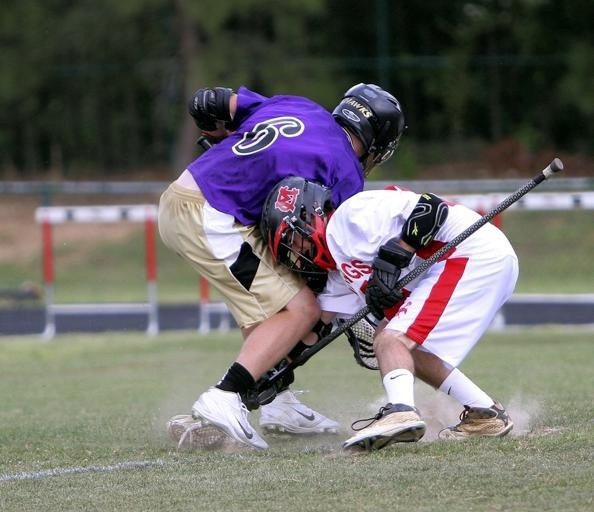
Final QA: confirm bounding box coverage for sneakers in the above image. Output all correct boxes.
[435,401,513,443]
[191,386,270,451]
[340,404,426,452]
[259,388,342,436]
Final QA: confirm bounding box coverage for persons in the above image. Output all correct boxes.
[259,177,519,452]
[157,83,404,452]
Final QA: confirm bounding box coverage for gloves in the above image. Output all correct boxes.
[364,238,412,321]
[240,358,294,410]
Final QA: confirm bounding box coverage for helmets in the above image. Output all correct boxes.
[260,176,334,285]
[332,82,407,176]
